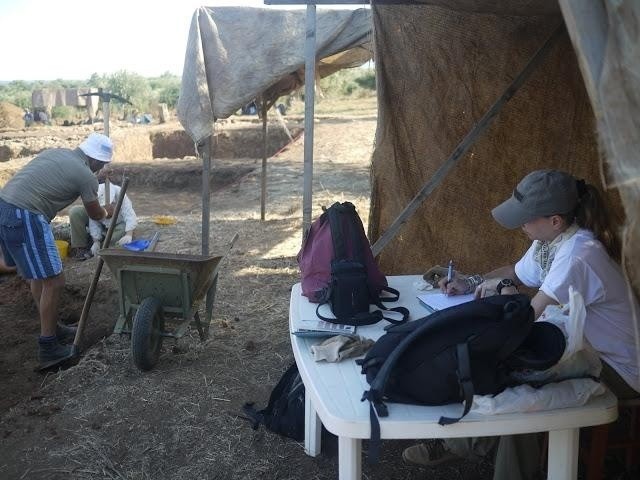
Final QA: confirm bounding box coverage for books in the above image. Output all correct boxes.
[417,292,475,314]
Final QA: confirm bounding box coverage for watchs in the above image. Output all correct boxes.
[496,278,518,295]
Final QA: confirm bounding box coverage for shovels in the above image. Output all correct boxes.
[34,178,129,373]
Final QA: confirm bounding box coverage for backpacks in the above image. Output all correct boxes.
[251,361,305,440]
[297,201,409,326]
[356,294,566,426]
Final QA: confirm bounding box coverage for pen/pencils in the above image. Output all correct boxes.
[445,259,453,298]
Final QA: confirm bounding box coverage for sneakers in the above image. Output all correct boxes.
[402,440,456,467]
[37,325,78,366]
[73,248,88,261]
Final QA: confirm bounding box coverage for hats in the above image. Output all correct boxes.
[79,133,114,163]
[98,183,115,206]
[491,169,579,230]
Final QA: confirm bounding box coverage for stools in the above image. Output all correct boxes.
[536,395,640,479]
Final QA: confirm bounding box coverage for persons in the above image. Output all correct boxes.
[404,168,640,469]
[0,132,114,363]
[69,182,137,260]
[23,108,34,127]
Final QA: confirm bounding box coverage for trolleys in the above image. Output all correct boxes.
[97,229,242,373]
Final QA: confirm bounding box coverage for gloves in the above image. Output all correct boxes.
[117,231,133,245]
[90,241,101,256]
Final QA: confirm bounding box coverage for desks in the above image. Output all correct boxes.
[288,270,619,480]
